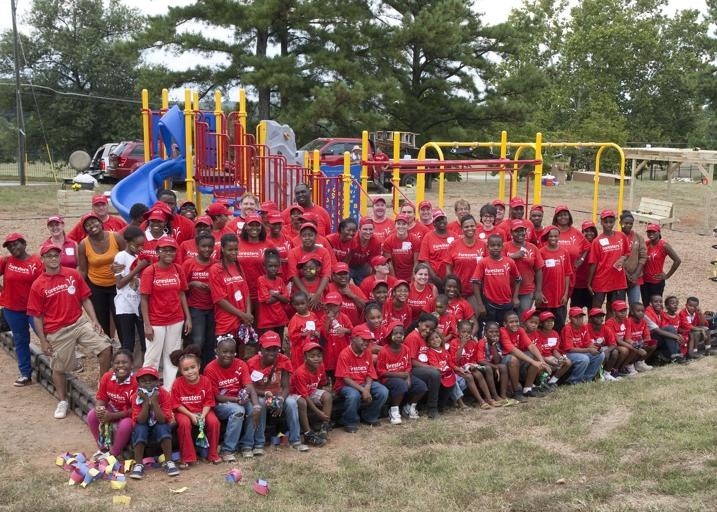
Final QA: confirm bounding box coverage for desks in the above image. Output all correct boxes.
[57,190,95,218]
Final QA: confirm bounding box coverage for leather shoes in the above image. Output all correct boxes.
[344,426,356,433]
[291,443,309,451]
[224,448,264,461]
[369,421,381,426]
[428,407,438,418]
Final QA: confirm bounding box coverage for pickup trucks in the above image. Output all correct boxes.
[300,137,440,192]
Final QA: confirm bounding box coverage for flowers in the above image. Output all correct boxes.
[72,183,81,188]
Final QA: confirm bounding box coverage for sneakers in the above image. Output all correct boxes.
[388,406,401,424]
[510,383,558,402]
[162,460,180,475]
[401,402,419,419]
[318,423,328,440]
[75,363,83,373]
[304,431,327,448]
[14,377,32,387]
[130,464,146,478]
[55,399,68,419]
[594,360,653,383]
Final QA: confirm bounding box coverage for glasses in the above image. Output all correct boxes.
[46,253,60,258]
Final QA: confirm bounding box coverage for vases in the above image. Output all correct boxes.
[73,187,80,191]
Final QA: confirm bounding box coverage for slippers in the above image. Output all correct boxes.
[461,398,519,412]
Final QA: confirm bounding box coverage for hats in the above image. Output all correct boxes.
[440,367,456,388]
[259,331,281,349]
[40,244,62,256]
[600,209,614,220]
[180,197,233,228]
[371,255,409,291]
[359,196,446,225]
[383,321,404,343]
[582,220,594,231]
[521,309,536,323]
[303,342,323,353]
[297,253,349,306]
[351,324,373,339]
[246,201,317,236]
[48,195,108,227]
[643,339,656,347]
[492,197,570,242]
[646,224,660,232]
[131,201,178,271]
[539,311,556,322]
[568,300,627,317]
[4,233,23,246]
[136,367,160,381]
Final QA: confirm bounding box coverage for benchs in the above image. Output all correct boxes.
[104,189,179,214]
[631,197,674,230]
[367,194,394,207]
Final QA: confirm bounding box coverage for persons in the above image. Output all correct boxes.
[350,145,362,165]
[171,144,179,158]
[372,148,389,194]
[1,184,711,479]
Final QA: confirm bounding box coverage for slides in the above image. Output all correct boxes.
[110,155,186,223]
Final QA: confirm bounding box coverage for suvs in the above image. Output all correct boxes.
[109,140,234,188]
[86,142,119,181]
